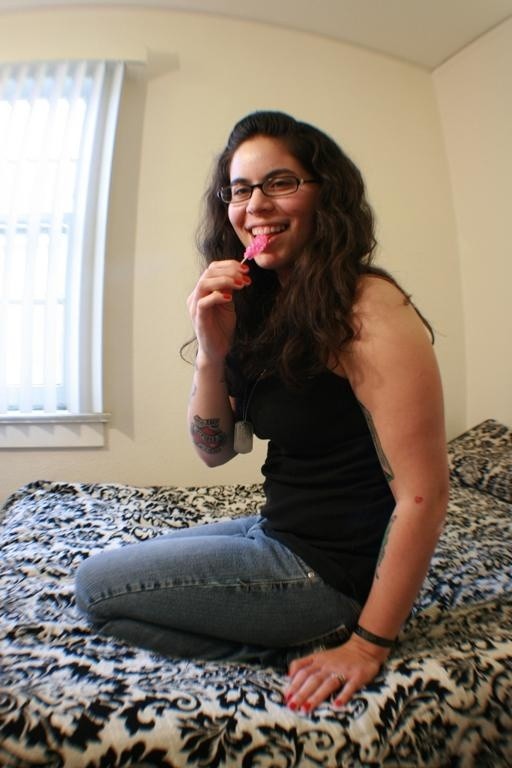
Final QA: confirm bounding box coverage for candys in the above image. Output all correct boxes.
[243,235,266,261]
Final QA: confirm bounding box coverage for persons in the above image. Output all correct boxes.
[73,111,450,713]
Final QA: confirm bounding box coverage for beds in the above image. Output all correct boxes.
[0,462,512,768]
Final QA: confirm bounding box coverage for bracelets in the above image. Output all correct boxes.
[352,625,396,648]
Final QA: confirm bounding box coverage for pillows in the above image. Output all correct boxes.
[445,414,510,506]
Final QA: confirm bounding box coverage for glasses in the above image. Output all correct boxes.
[217,174,313,204]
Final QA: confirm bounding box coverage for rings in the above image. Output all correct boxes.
[333,671,344,683]
